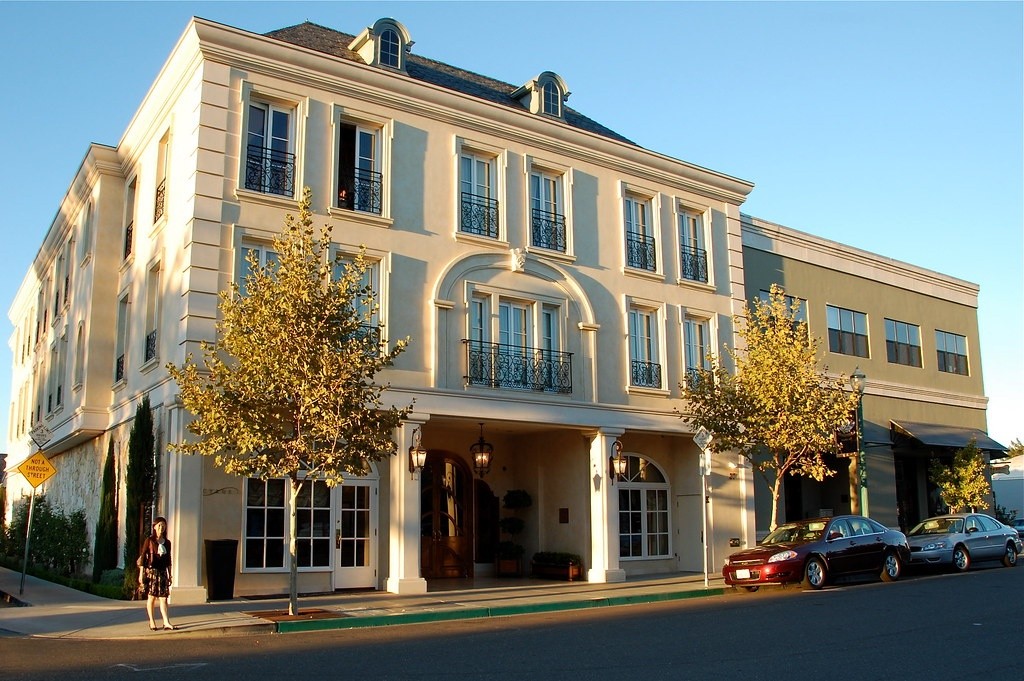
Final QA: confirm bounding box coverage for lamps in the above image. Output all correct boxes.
[609,441,627,486]
[470,423,494,480]
[408,427,427,479]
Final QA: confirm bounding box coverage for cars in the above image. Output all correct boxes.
[722,514,911,594]
[1007,517,1024,537]
[904,512,1024,575]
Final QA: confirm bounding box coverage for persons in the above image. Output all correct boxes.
[136,517,178,631]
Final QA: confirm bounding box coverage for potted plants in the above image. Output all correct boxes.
[532,550,583,582]
[495,487,532,577]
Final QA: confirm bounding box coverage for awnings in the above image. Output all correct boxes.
[831,419,1011,453]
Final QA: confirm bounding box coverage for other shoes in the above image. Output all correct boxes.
[149,623,157,631]
[163,623,178,630]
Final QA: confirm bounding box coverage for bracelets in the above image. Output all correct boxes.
[168,576,172,578]
